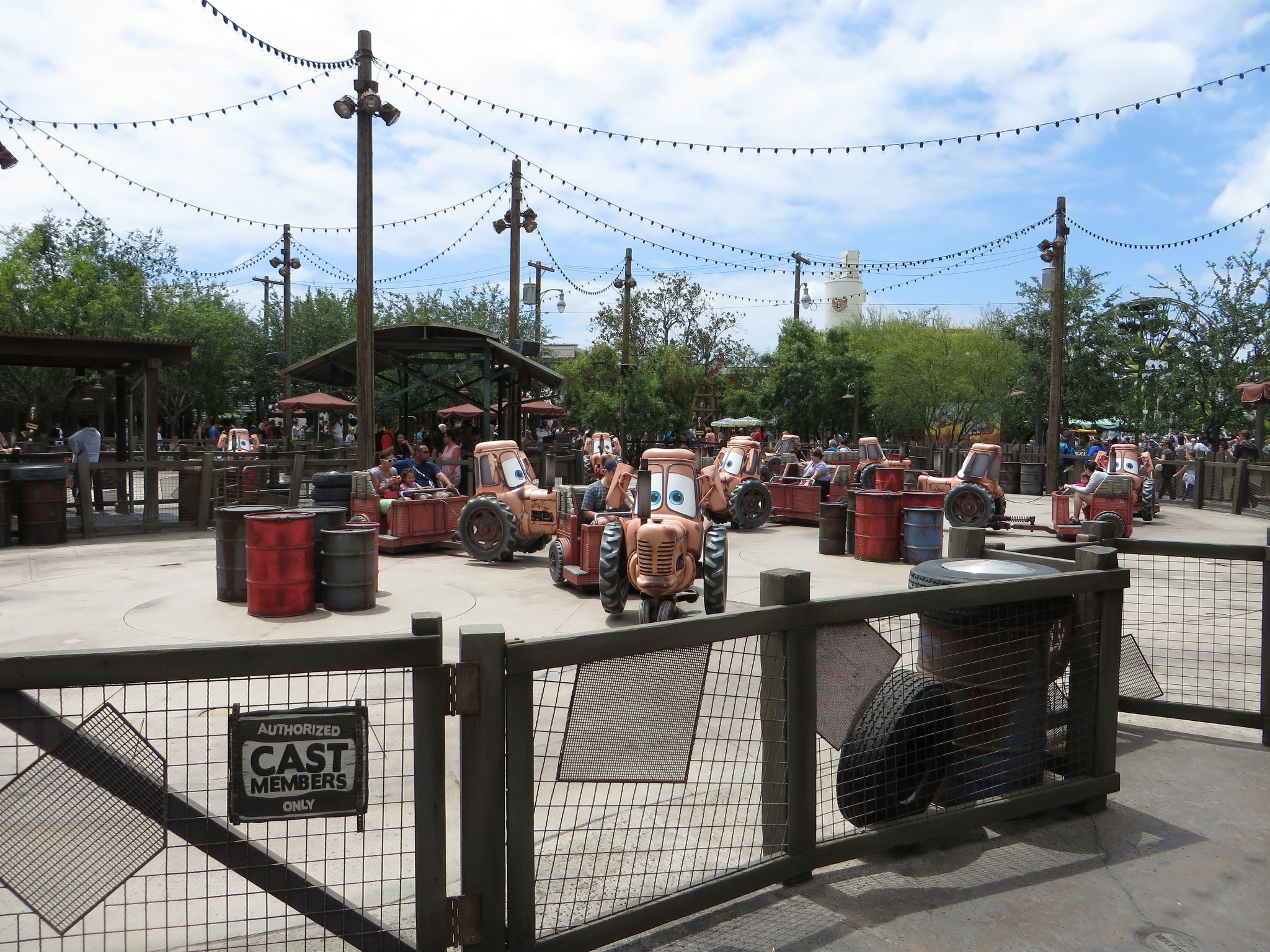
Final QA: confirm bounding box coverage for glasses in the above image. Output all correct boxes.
[1237,435,1244,437]
[416,450,430,454]
[443,434,449,438]
[470,432,479,436]
[381,457,395,464]
[1161,444,1166,447]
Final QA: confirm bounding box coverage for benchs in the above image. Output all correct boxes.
[1089,471,1133,525]
[821,450,859,479]
[783,461,850,502]
[351,468,444,535]
[780,452,807,475]
[561,483,634,569]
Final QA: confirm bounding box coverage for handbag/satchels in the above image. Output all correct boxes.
[382,490,399,500]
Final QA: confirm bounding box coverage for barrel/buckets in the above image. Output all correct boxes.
[0,479,11,548]
[908,455,928,470]
[855,492,903,562]
[898,490,945,558]
[875,467,905,491]
[903,506,944,566]
[1020,463,1045,496]
[998,462,1021,494]
[320,525,377,611]
[691,447,707,456]
[819,501,847,556]
[243,512,317,617]
[887,453,909,460]
[17,479,67,546]
[346,521,380,593]
[917,611,1055,820]
[282,505,348,604]
[214,505,283,603]
[712,450,721,456]
[904,469,934,491]
[845,489,892,555]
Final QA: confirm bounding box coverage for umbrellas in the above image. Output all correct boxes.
[434,396,569,442]
[264,391,358,443]
[711,415,767,443]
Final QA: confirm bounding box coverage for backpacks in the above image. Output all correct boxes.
[373,429,396,458]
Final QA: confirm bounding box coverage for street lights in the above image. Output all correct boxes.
[790,252,811,324]
[332,30,405,477]
[1038,193,1072,497]
[269,222,301,471]
[492,160,539,456]
[612,248,638,471]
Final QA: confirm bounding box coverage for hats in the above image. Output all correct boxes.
[445,422,452,428]
[427,425,440,433]
[539,420,549,427]
[470,427,481,433]
[603,459,620,473]
[494,425,498,431]
[325,414,338,422]
[569,427,579,432]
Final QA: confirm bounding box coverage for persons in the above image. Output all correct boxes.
[374,412,717,458]
[580,459,638,525]
[813,431,918,457]
[718,424,773,450]
[370,449,412,535]
[222,415,358,450]
[209,420,222,439]
[45,416,163,516]
[399,467,434,500]
[363,442,455,500]
[789,441,805,463]
[196,419,208,447]
[798,448,831,503]
[1013,429,1270,524]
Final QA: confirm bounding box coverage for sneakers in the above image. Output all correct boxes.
[1068,518,1081,527]
[1069,516,1073,521]
